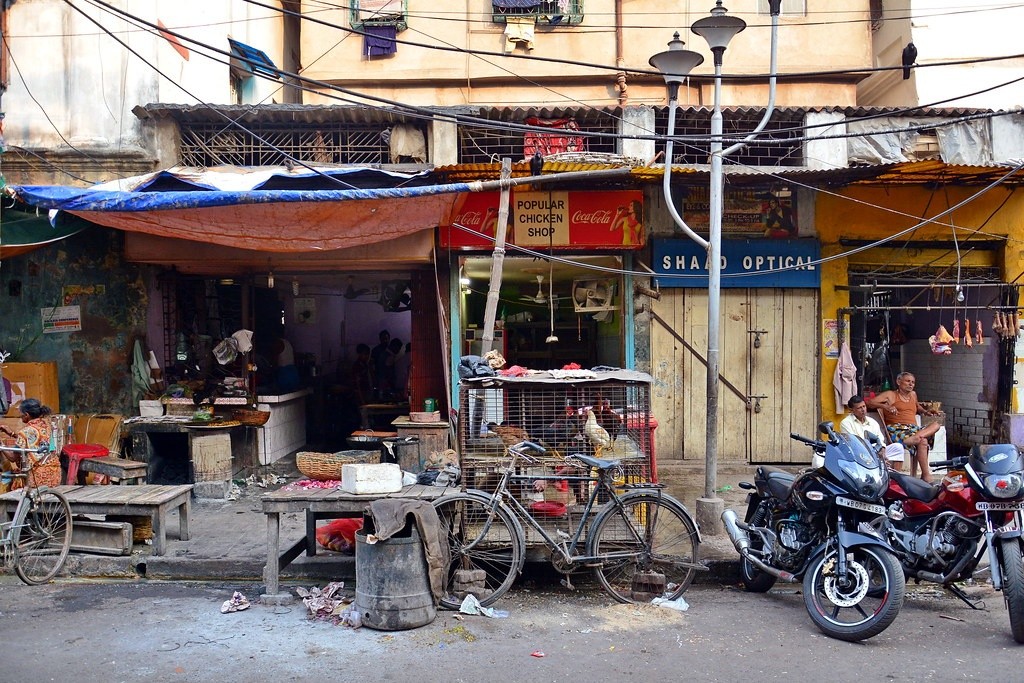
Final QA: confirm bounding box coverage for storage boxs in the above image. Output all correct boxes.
[341,463,402,495]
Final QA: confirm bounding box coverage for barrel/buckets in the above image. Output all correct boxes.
[354,508,437,631]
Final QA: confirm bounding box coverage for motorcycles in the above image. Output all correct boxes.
[853,443,1024,647]
[719,418,916,644]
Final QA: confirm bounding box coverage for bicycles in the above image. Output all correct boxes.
[429,436,709,612]
[0,441,73,587]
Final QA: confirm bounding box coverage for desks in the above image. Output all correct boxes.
[0,485,194,555]
[260,475,462,594]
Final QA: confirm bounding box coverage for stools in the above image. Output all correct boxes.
[61,443,110,484]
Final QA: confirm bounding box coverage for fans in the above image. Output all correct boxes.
[307,275,383,299]
[517,275,572,304]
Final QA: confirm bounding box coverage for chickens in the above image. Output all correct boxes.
[485,398,622,516]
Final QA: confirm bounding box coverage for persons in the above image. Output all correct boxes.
[840,395,903,471]
[351,329,411,431]
[866,372,941,483]
[0,375,11,414]
[253,325,295,396]
[533,399,622,492]
[0,398,62,491]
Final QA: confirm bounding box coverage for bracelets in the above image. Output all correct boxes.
[10,432,14,438]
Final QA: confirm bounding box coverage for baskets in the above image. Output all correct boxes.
[105,515,153,544]
[296,451,357,481]
[231,408,271,426]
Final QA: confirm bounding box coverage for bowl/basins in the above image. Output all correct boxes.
[530,500,567,516]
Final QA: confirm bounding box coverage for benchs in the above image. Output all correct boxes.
[59,452,148,485]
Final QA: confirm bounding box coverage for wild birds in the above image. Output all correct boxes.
[902,43,918,80]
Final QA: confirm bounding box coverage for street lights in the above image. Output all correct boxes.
[643,1,786,537]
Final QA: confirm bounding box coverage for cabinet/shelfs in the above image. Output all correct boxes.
[512,321,596,369]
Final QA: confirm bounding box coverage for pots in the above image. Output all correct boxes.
[346,429,384,451]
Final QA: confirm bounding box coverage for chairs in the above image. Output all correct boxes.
[876,409,918,477]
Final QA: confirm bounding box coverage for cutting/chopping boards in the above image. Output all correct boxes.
[409,411,440,423]
